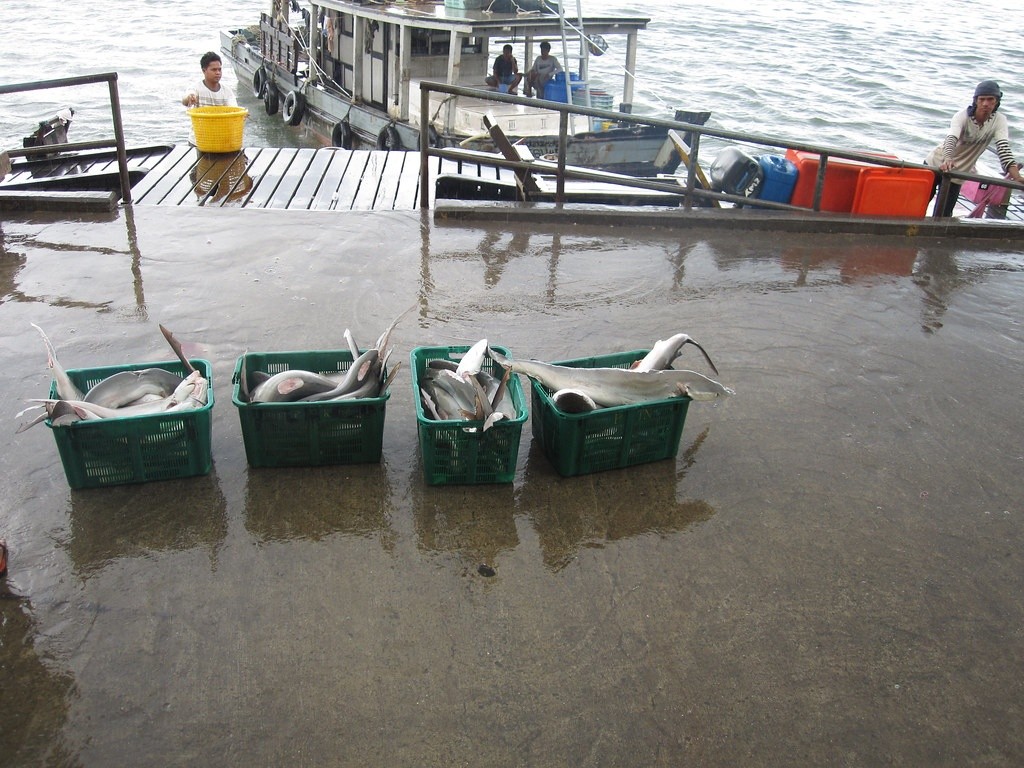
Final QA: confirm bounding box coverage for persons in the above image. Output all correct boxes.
[524,42,563,98]
[182,52,248,147]
[485,45,522,95]
[923,81,1024,203]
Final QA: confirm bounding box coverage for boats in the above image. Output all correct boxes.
[216,0,712,176]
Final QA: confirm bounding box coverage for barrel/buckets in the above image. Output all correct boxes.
[747,152,798,209]
[541,72,585,102]
[711,147,763,196]
[572,88,613,111]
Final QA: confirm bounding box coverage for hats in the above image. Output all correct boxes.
[973,81,1001,98]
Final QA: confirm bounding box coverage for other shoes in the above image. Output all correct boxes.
[508,90,518,95]
[527,91,532,98]
[488,89,497,92]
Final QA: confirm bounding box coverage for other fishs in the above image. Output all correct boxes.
[241,302,419,457]
[15,319,209,487]
[421,333,733,477]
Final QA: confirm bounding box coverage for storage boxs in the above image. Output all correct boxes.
[44,358,213,489]
[232,351,391,467]
[528,349,692,479]
[407,345,530,486]
